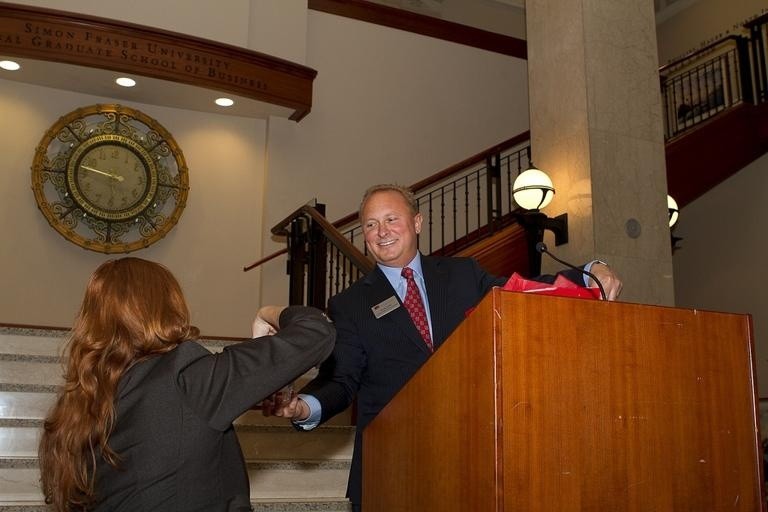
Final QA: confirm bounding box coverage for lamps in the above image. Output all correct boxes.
[512,161,569,247]
[667,194,684,248]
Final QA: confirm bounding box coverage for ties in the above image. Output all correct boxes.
[401,267,433,353]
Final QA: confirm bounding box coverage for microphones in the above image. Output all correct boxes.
[537,242,606,301]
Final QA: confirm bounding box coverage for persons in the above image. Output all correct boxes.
[35,257,337,512]
[263,183,625,512]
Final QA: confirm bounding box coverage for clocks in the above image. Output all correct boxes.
[29,103,190,255]
